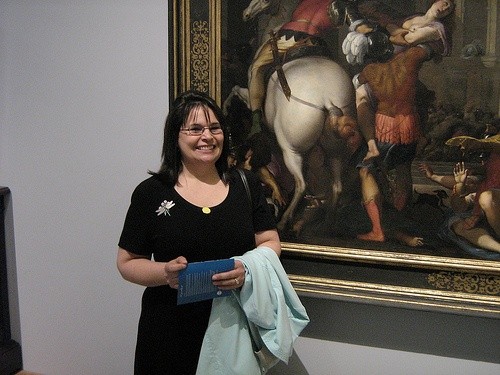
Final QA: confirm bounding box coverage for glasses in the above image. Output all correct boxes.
[179,121,223,135]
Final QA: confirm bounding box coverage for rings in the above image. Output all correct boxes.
[235,279,239,285]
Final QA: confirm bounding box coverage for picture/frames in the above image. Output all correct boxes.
[168,0,500,319]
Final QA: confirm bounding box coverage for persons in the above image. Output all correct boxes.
[117,90,282,375]
